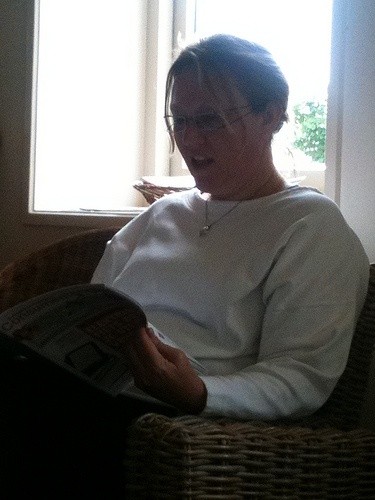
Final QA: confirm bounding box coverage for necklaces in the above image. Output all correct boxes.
[197,166,278,238]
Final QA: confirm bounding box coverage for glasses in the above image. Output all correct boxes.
[164,106,251,133]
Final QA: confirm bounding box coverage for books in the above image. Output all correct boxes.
[0,281,207,400]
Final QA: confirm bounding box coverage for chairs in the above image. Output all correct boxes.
[0,227,375,500]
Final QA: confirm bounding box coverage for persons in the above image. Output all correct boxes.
[0,34,375,500]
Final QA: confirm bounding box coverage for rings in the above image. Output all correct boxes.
[126,358,145,371]
